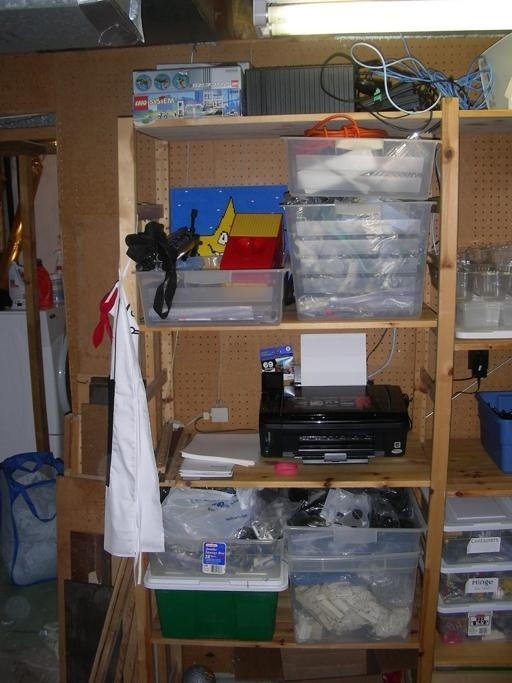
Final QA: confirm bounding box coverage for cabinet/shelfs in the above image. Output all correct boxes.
[428,99,510,677]
[119,97,453,678]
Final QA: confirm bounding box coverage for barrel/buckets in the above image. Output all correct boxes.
[48,273,65,307]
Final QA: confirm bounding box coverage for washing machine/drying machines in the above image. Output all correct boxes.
[0,307,72,462]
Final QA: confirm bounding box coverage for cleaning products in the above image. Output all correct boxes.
[36,259,52,309]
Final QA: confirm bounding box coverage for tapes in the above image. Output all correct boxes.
[273,462,299,476]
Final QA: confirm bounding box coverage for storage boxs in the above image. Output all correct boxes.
[273,489,428,552]
[472,388,512,476]
[278,194,439,325]
[285,548,425,643]
[435,591,509,646]
[146,528,279,579]
[131,259,293,328]
[439,495,511,562]
[438,557,509,602]
[275,129,442,202]
[142,560,290,642]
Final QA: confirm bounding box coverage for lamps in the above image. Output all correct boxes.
[252,0,512,39]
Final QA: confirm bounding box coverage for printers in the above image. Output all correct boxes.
[258,370,408,465]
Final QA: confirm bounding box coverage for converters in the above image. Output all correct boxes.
[474,360,486,377]
